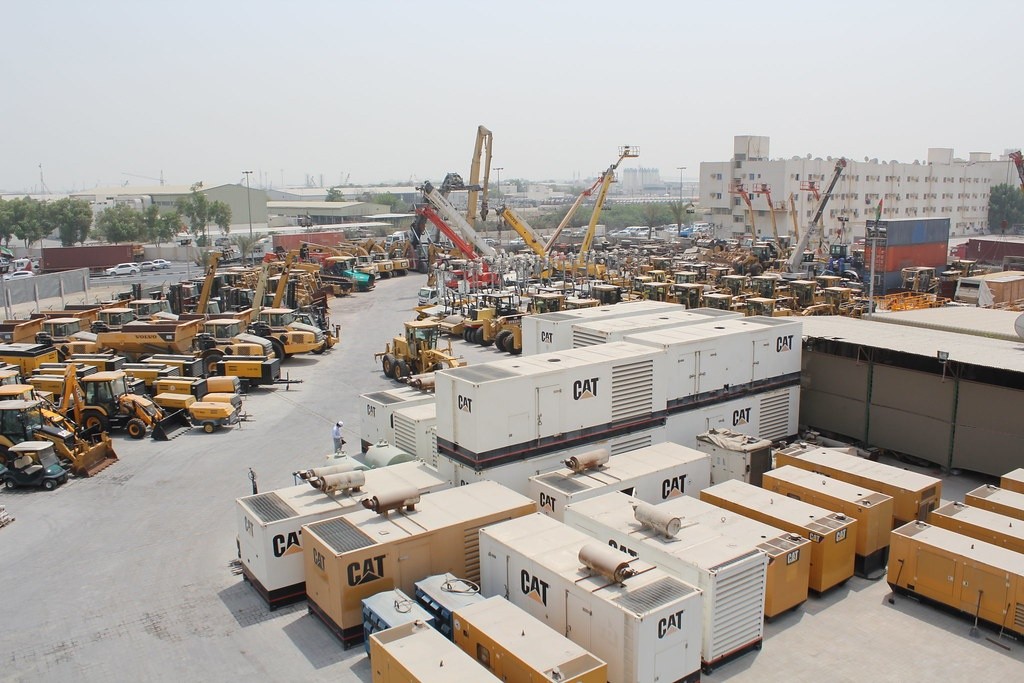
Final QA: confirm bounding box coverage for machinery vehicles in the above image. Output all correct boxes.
[0,124,985,492]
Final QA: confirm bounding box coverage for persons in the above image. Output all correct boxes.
[333,421,344,453]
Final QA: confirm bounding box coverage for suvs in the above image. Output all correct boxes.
[2,270,35,280]
[0,256,9,274]
[105,261,140,275]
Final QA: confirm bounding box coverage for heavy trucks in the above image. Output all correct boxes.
[949,238,1024,264]
[11,243,134,271]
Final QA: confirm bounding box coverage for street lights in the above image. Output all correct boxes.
[242,170,254,238]
[675,167,687,231]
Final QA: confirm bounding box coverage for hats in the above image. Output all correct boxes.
[338,421,344,427]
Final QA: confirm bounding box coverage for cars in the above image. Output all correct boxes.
[153,259,171,269]
[137,260,161,270]
[484,222,710,245]
